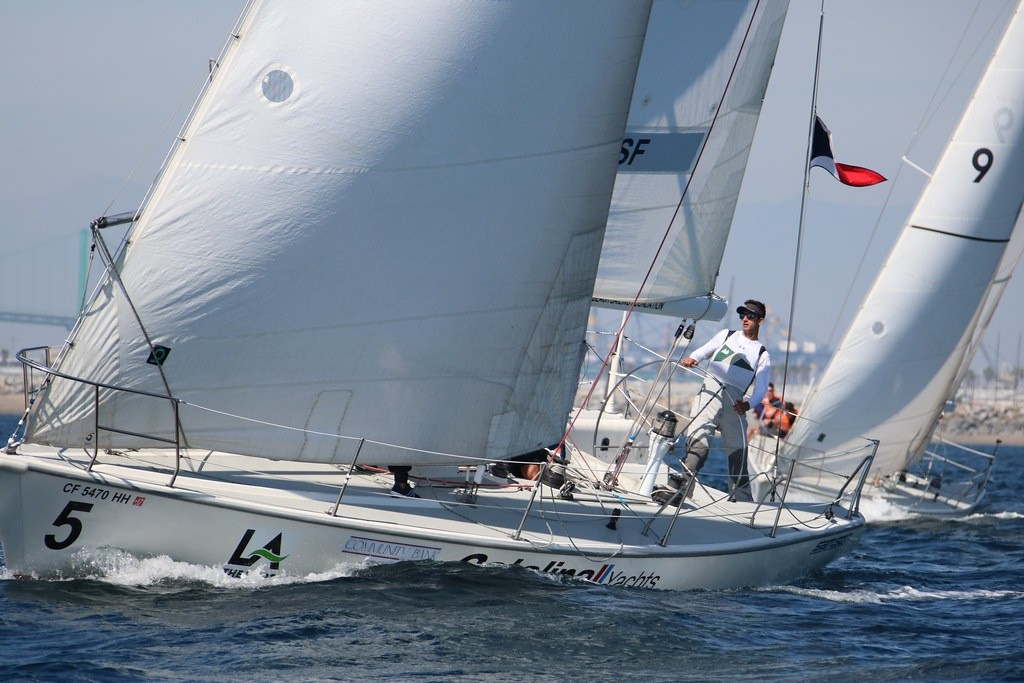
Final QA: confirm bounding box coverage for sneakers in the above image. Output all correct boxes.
[391,483,420,500]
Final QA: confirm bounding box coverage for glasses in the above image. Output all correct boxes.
[766,390,772,392]
[740,312,759,320]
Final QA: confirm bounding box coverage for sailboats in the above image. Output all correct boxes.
[556,0,1024,538]
[0,1,880,595]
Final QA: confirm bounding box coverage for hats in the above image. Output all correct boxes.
[736,299,766,318]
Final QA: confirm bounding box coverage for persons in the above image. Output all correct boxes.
[388,465,421,499]
[679,299,771,503]
[761,382,798,439]
[507,443,567,480]
